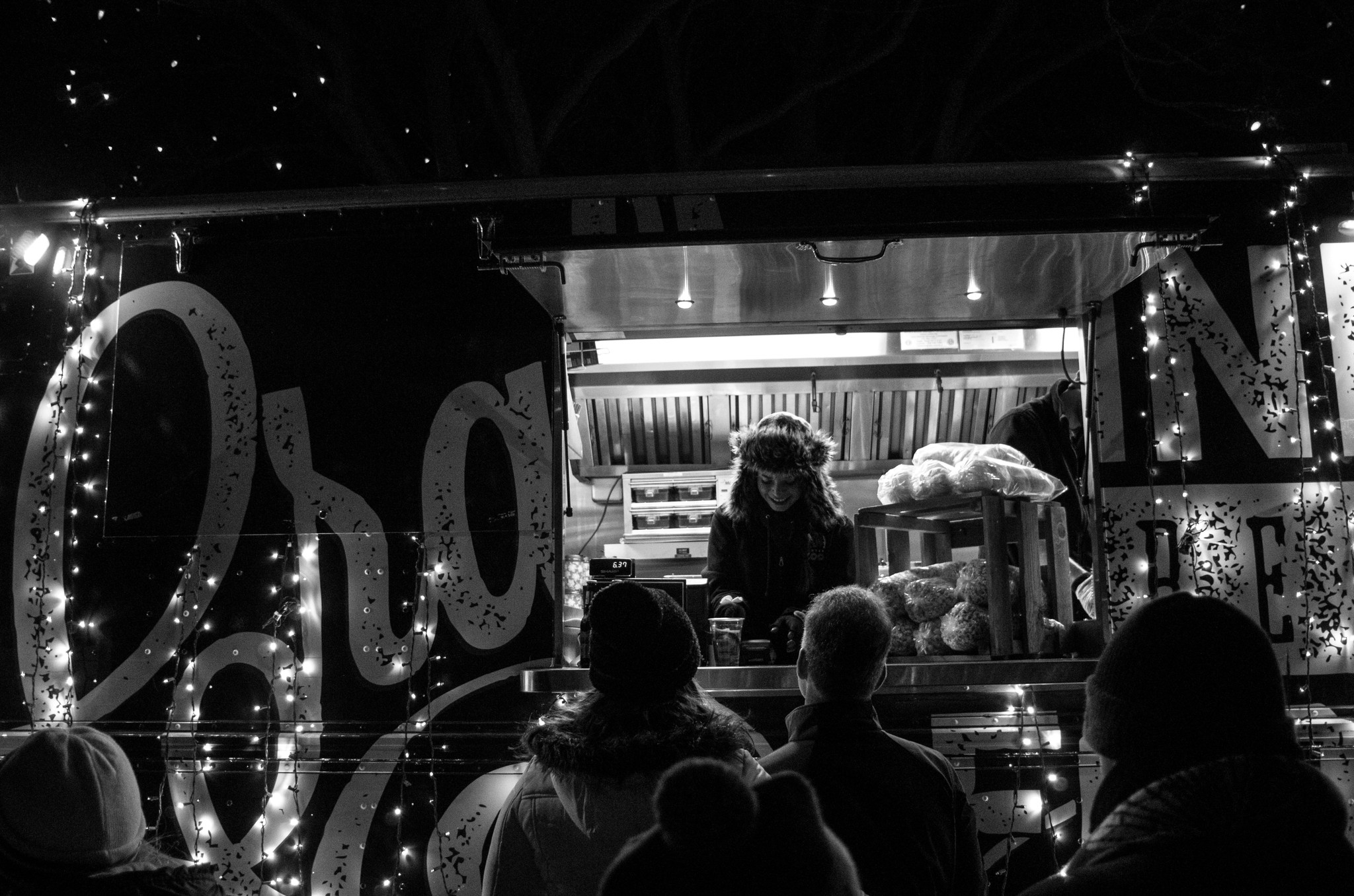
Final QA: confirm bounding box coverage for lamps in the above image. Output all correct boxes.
[675,246,694,309]
[964,237,984,300]
[820,241,839,306]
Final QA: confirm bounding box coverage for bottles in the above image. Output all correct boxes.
[584,556,591,585]
[564,554,583,589]
[564,589,584,608]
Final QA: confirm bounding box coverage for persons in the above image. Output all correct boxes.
[482,577,867,896]
[1031,590,1354,896]
[985,370,1096,622]
[704,409,856,662]
[751,584,993,896]
[598,753,870,896]
[1,726,228,896]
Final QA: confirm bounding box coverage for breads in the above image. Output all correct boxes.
[878,443,1056,505]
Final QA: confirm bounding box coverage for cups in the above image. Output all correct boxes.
[708,618,745,667]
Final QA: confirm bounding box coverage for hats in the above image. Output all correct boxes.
[1084,591,1300,766]
[589,581,701,698]
[597,761,862,896]
[724,411,847,530]
